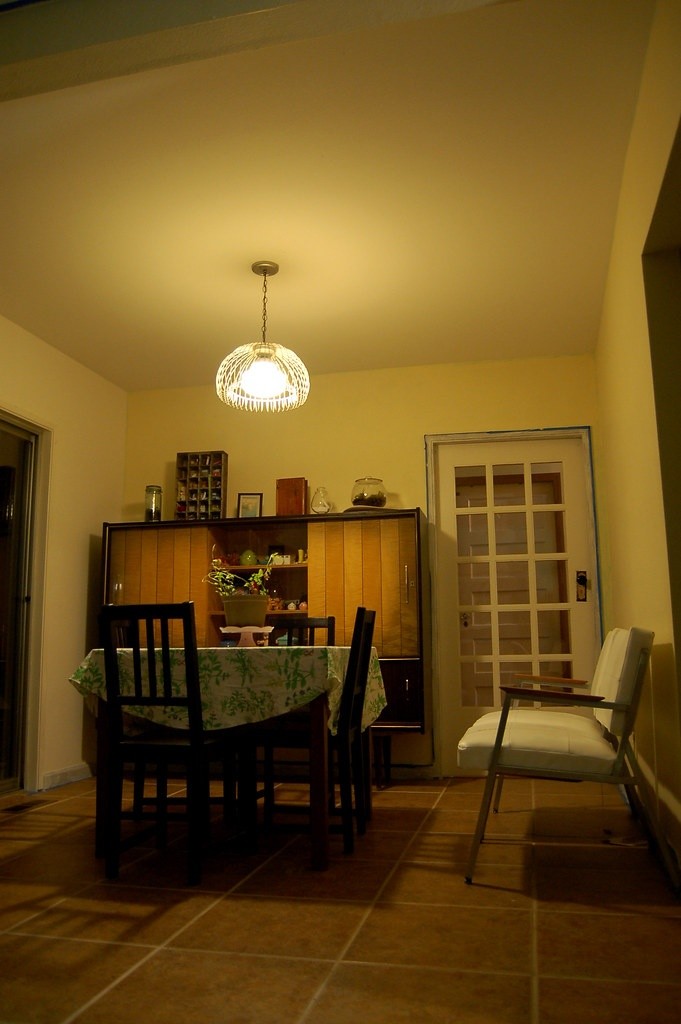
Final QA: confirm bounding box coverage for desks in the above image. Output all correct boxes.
[68,644,391,859]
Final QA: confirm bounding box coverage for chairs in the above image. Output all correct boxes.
[98,601,375,883]
[455,626,681,894]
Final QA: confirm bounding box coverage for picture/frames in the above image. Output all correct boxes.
[237,492,263,518]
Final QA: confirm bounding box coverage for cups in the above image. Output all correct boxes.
[145,484,162,523]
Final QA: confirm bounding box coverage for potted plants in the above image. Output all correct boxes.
[200,544,279,628]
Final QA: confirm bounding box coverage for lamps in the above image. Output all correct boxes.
[215,261,311,413]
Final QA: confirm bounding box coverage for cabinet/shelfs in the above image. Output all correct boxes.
[174,451,228,521]
[99,507,435,735]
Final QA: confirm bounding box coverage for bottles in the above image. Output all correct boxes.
[311,485,331,513]
